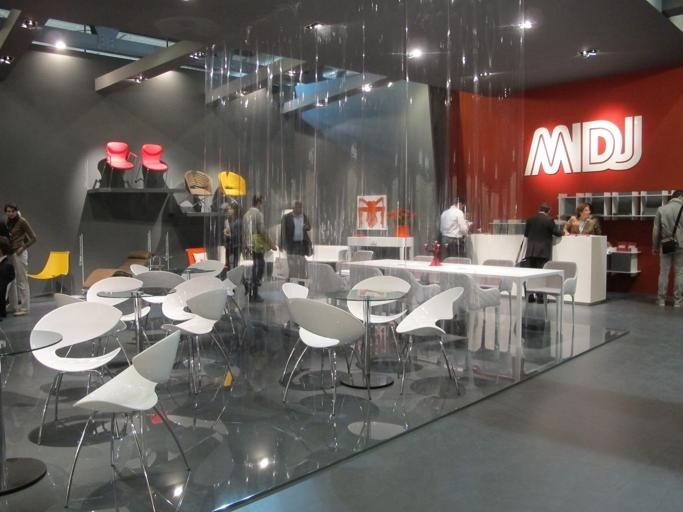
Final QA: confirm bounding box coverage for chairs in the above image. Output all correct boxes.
[183,169,248,211]
[99,140,169,187]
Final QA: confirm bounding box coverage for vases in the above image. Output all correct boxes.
[393,223,410,237]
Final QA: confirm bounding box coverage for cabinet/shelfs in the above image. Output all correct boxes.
[86,186,230,219]
[606,251,643,278]
[556,189,673,221]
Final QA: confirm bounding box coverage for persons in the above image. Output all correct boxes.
[223,202,242,276]
[4,200,37,316]
[0,235,13,322]
[523,203,565,304]
[463,210,472,222]
[650,190,682,308]
[564,203,603,237]
[437,197,467,259]
[237,191,278,302]
[277,201,313,285]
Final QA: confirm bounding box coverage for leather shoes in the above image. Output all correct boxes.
[14,311,28,315]
[528,295,535,302]
[5,306,18,313]
[537,297,549,303]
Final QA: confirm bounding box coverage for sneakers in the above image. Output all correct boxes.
[655,300,665,306]
[251,294,264,303]
[673,303,682,308]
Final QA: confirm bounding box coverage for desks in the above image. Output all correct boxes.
[0,328,66,496]
[345,234,414,260]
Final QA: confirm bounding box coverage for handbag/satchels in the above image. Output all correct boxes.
[247,234,270,254]
[304,232,313,257]
[520,258,529,267]
[662,239,675,254]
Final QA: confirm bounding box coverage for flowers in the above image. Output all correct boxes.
[388,208,417,227]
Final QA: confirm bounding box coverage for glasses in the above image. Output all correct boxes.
[584,211,590,214]
